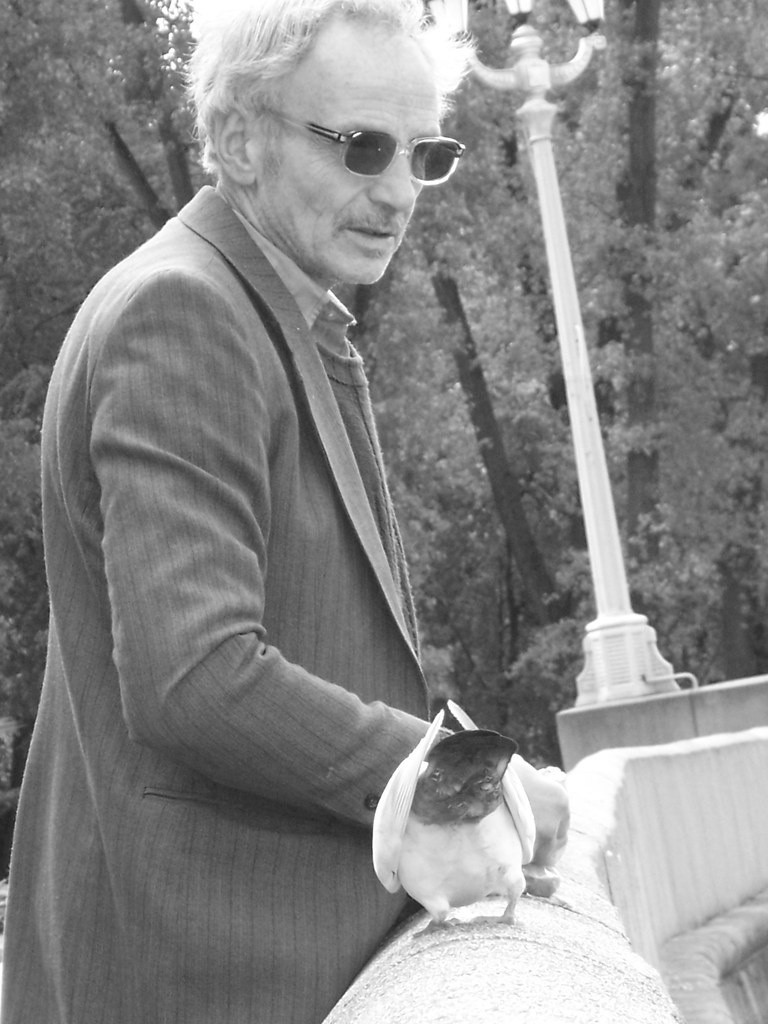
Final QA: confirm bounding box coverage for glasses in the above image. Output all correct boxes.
[261,108,467,186]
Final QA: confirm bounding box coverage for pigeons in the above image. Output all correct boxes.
[373,700,537,938]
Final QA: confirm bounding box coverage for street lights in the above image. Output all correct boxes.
[448,0,679,704]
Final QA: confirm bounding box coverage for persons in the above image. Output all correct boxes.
[0,0,573,1024]
